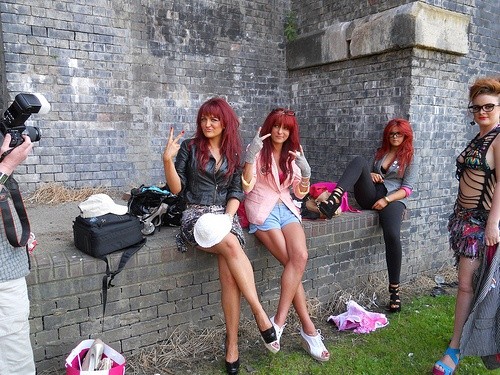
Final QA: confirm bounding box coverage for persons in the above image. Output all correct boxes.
[163,98,277,375]
[0,131,38,375]
[434,77,500,375]
[316,119,419,313]
[241,108,330,362]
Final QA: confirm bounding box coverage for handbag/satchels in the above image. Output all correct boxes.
[129,182,185,227]
[65,339,125,375]
[309,183,360,213]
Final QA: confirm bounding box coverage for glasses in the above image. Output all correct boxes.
[388,132,403,138]
[272,107,296,116]
[467,103,500,113]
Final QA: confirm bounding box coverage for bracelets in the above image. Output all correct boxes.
[0,172,9,186]
[301,183,309,187]
[301,179,308,183]
[384,197,390,203]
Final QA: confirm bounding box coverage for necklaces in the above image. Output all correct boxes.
[215,157,222,171]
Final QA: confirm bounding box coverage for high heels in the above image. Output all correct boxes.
[318,186,345,219]
[225,334,240,375]
[82,338,104,371]
[299,327,329,361]
[261,315,287,354]
[387,284,401,313]
[254,307,277,344]
[96,358,112,370]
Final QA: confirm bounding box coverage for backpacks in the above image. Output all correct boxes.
[72,213,147,276]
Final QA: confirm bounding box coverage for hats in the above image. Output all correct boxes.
[193,213,232,248]
[78,192,128,219]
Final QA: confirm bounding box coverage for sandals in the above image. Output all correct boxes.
[432,345,461,375]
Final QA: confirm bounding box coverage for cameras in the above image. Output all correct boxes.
[0,93,50,147]
